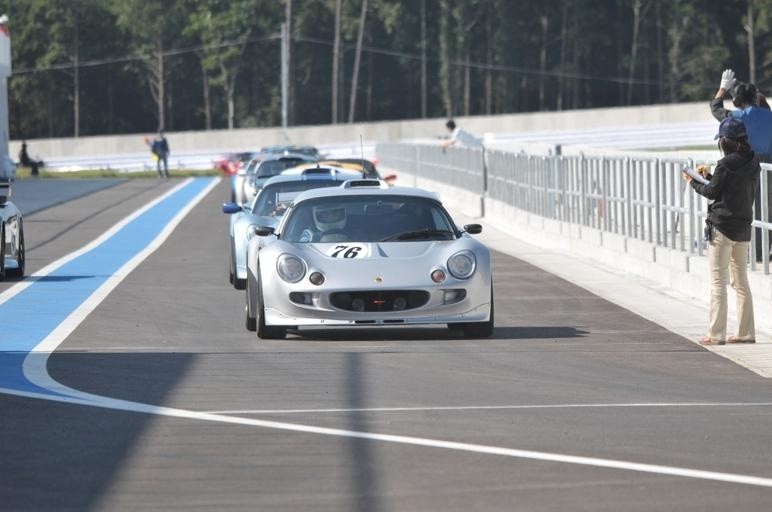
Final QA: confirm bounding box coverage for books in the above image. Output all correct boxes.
[683,166,710,185]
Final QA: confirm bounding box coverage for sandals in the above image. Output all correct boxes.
[699,335,726,345]
[728,335,756,343]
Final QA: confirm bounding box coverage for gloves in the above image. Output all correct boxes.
[720,69,738,91]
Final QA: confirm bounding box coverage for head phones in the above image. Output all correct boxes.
[733,84,743,107]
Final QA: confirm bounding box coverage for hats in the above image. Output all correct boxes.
[714,117,748,139]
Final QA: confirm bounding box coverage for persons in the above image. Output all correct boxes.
[681,117,761,345]
[438,119,485,151]
[709,69,771,262]
[147,127,170,179]
[18,143,39,176]
[753,89,769,111]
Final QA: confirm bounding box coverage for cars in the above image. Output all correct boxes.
[1,177,31,279]
